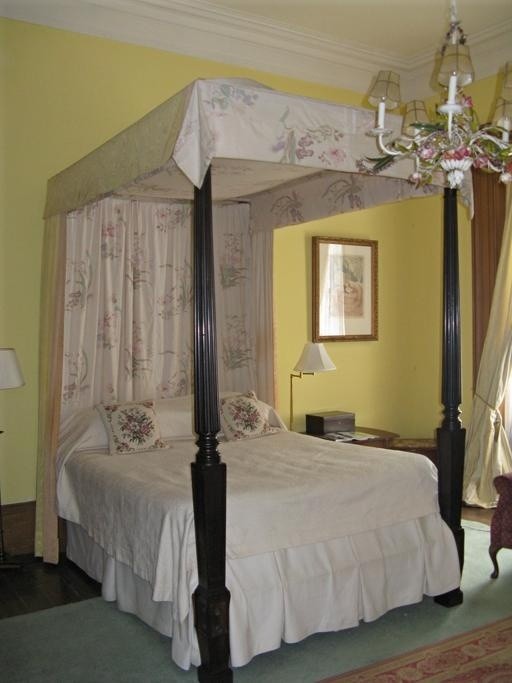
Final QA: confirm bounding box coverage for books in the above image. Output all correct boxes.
[325,431,380,442]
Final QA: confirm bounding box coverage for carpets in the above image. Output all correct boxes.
[322,616,512,683]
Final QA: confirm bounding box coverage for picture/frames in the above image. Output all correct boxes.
[312,236,378,345]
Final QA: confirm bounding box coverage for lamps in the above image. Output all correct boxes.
[289,343,335,431]
[0,347,26,390]
[356,0,511,190]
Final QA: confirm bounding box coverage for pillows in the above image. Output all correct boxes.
[219,391,277,440]
[104,398,167,456]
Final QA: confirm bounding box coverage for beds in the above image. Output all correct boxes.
[61,427,440,671]
